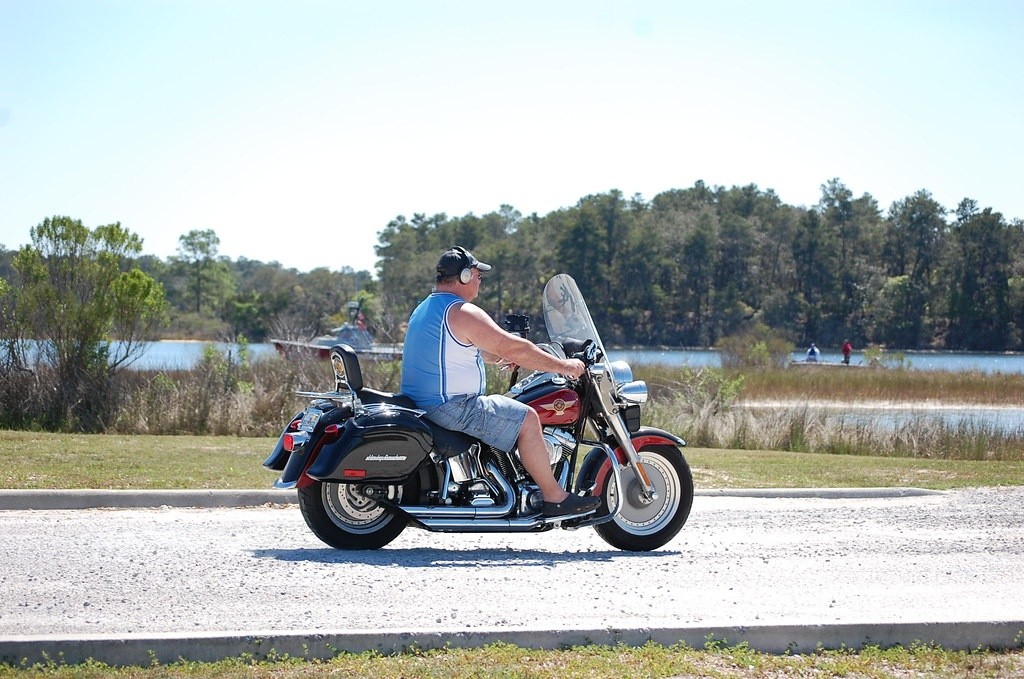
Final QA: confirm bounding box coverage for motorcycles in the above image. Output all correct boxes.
[261,273,694,550]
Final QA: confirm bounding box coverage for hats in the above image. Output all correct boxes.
[433,246,491,280]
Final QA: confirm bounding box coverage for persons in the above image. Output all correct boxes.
[841,338,852,364]
[400,246,602,519]
[805,343,820,362]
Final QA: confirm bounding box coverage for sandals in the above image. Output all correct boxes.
[542,493,601,518]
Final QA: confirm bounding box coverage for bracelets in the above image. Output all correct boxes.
[495,358,504,364]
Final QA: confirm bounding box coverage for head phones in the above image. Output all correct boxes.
[451,245,472,285]
